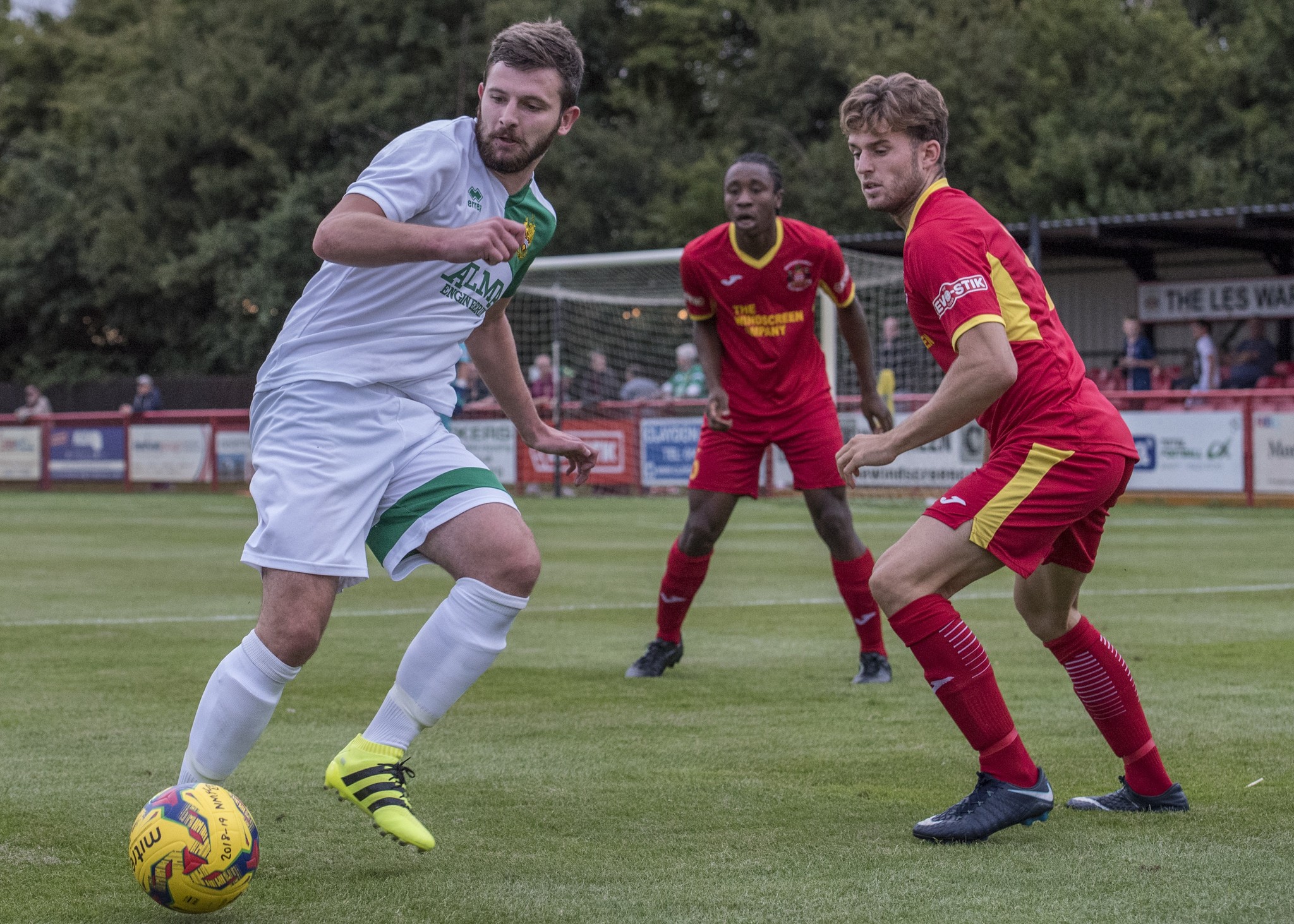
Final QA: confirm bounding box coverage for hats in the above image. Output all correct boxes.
[676,343,699,359]
[137,374,153,384]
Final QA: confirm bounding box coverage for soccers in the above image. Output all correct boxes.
[128,782,261,914]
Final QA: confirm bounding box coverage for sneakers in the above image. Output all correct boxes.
[1065,775,1189,812]
[624,635,683,679]
[324,733,435,855]
[912,766,1054,843]
[853,651,893,684]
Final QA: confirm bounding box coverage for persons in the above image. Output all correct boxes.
[830,71,1188,842]
[450,315,1294,409]
[119,373,163,418]
[13,384,54,423]
[621,153,898,686]
[177,15,586,849]
